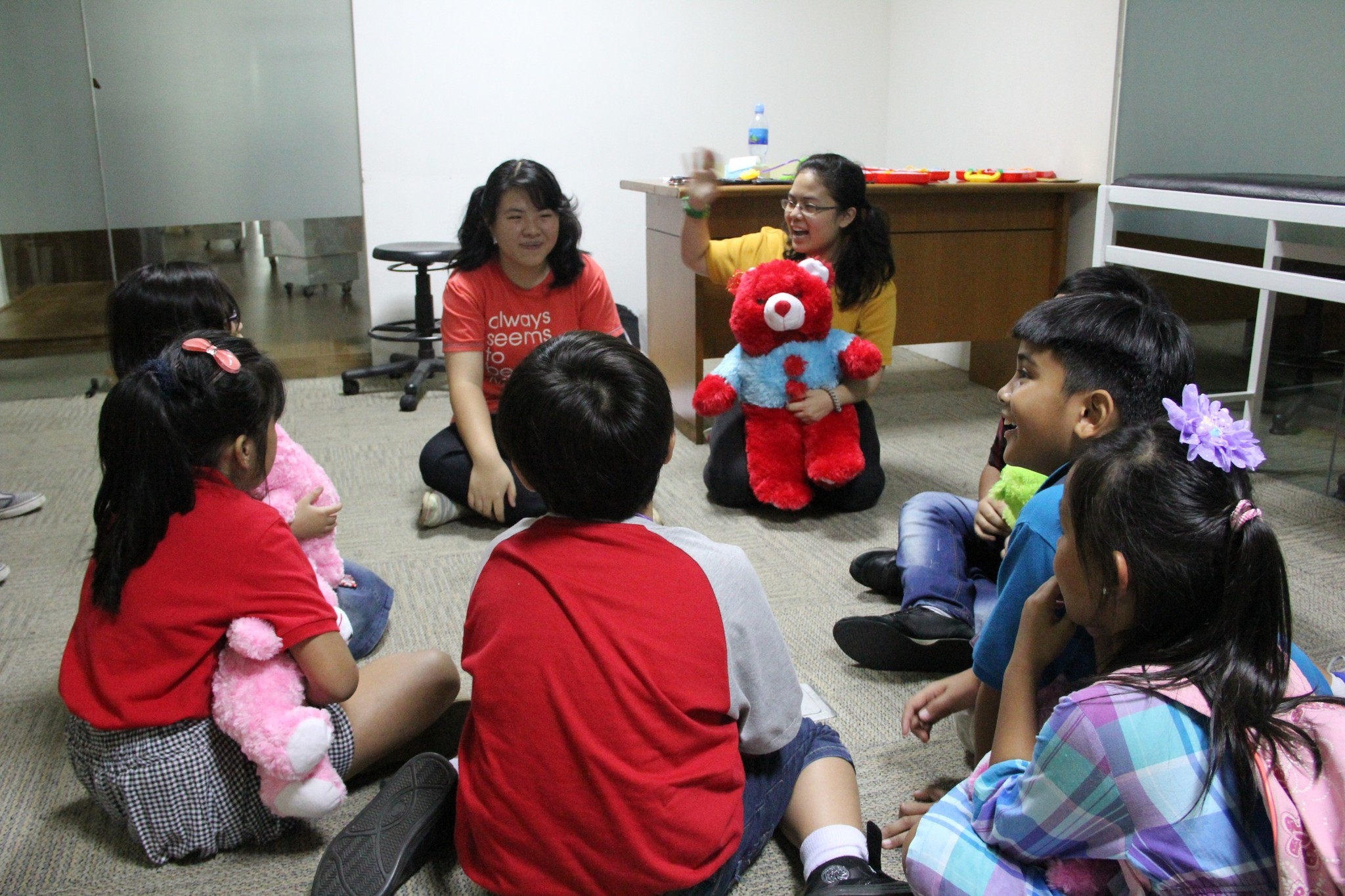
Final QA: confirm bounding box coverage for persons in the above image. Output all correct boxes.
[880,291,1335,858]
[829,262,1173,667]
[311,329,917,895]
[674,142,899,521]
[891,416,1344,896]
[55,327,461,862]
[417,153,631,529]
[106,260,394,655]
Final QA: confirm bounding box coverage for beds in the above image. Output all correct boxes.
[1089,169,1345,440]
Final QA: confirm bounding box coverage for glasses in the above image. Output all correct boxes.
[781,199,842,215]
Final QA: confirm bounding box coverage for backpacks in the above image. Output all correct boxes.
[1109,644,1344,895]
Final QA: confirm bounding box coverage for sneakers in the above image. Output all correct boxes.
[417,489,463,528]
[311,752,457,895]
[1,492,47,518]
[797,857,913,895]
[849,547,904,601]
[832,606,976,670]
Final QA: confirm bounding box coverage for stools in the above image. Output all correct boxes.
[340,240,463,411]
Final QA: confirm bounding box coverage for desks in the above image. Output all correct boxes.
[618,174,1101,447]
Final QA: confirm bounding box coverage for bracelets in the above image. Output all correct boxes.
[681,196,710,221]
[818,383,843,413]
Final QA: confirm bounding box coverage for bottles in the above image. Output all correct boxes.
[749,104,770,179]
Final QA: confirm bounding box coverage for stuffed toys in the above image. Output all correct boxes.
[691,255,886,510]
[990,464,1047,529]
